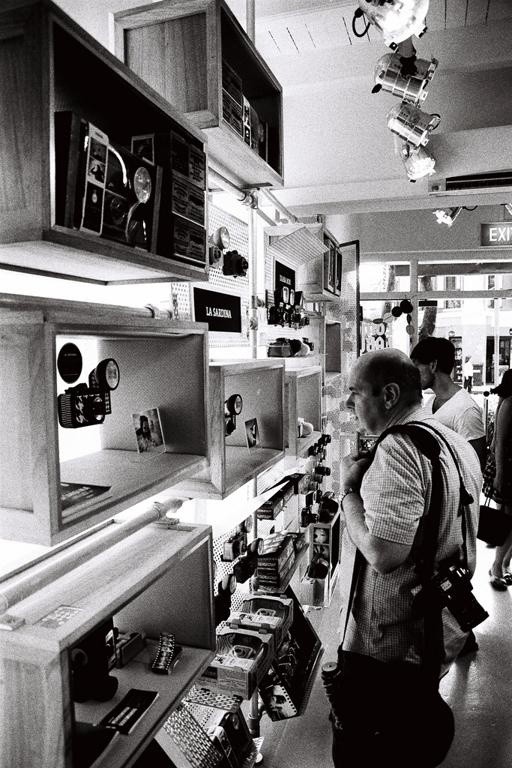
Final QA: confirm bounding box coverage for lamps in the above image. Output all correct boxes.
[346,0,441,182]
[433,202,468,227]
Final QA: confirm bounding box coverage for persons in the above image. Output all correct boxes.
[338,349,485,691]
[487,369,512,592]
[409,336,486,660]
[462,355,474,394]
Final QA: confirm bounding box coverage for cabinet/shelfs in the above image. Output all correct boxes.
[0,520,218,768]
[115,3,285,190]
[0,2,210,288]
[170,360,289,498]
[283,368,326,460]
[1,292,213,545]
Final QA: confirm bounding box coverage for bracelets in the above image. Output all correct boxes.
[339,487,355,512]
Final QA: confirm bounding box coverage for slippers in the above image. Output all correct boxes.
[488,570,511,591]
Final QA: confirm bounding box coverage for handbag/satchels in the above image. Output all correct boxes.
[322,648,455,768]
[482,447,511,504]
[476,503,511,548]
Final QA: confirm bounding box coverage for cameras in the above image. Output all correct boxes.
[224,250,248,279]
[255,607,277,617]
[228,643,253,658]
[268,286,309,330]
[434,554,489,633]
